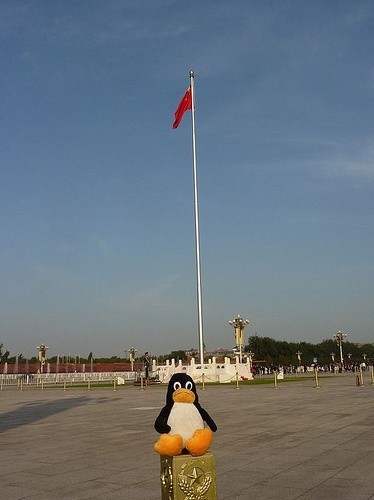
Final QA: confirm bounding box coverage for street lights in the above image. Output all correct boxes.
[128,347,137,372]
[296,351,303,367]
[36,343,49,374]
[228,314,249,363]
[330,352,336,362]
[362,353,367,361]
[346,352,353,359]
[333,330,348,371]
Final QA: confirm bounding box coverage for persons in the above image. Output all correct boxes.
[313,362,366,373]
[288,363,307,373]
[252,364,286,375]
[144,352,151,379]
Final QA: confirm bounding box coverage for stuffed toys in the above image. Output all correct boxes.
[153,373,218,456]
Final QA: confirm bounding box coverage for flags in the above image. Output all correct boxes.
[172,87,192,129]
